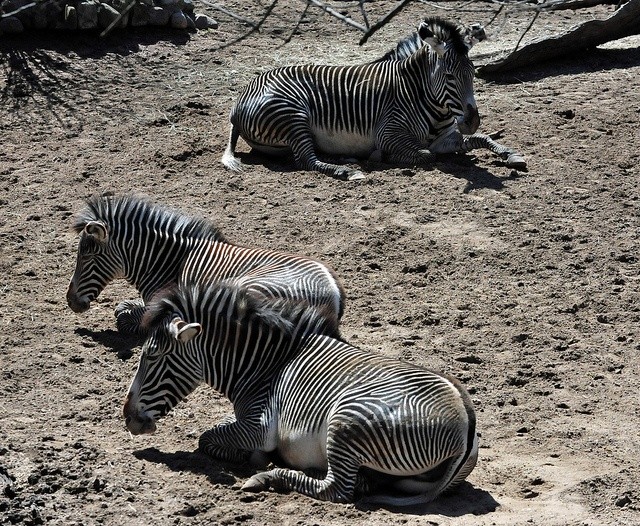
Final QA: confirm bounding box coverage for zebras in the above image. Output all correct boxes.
[123,277,479,506]
[220,15,527,181]
[66,190,346,334]
